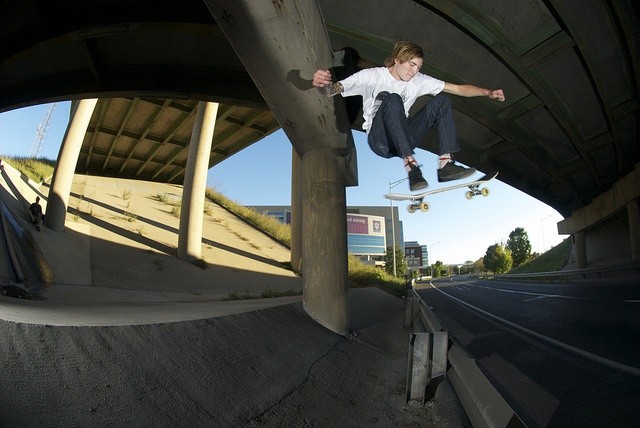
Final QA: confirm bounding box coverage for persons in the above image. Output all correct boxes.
[28,196,42,232]
[312,42,505,191]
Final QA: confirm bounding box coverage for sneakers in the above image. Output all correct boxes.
[407,163,427,191]
[437,162,476,182]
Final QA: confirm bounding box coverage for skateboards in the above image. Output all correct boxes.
[384,170,499,213]
